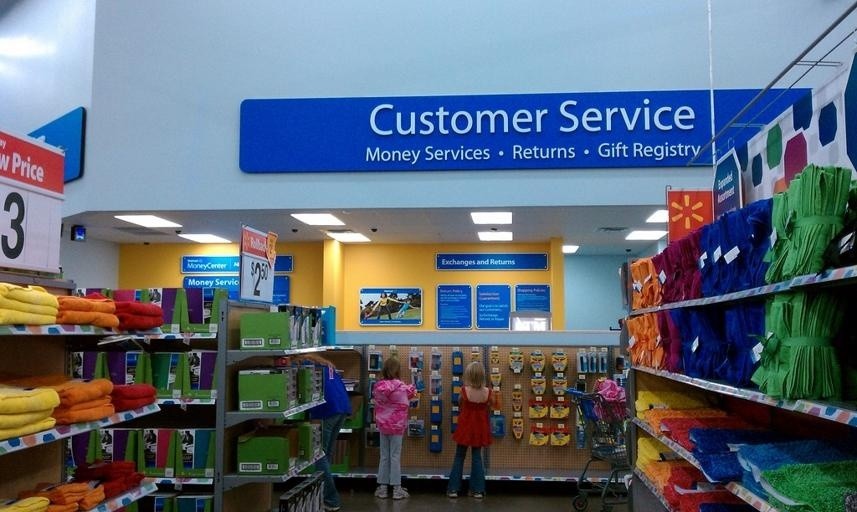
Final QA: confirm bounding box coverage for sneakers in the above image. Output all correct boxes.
[324,503,341,511]
[473,492,483,497]
[447,490,457,497]
[375,486,388,498]
[393,488,409,499]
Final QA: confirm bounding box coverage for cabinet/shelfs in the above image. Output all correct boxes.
[0,281,213,510]
[215,292,366,511]
[620,248,857,511]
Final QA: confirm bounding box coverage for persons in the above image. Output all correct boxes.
[371,358,417,499]
[295,353,352,511]
[361,292,419,320]
[446,362,494,499]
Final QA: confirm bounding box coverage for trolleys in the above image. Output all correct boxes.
[562,386,632,512]
